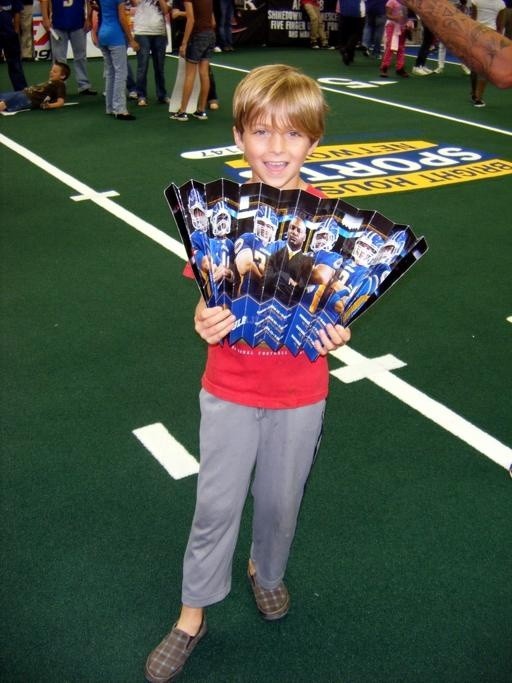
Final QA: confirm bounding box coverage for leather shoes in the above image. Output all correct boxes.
[113,113,136,120]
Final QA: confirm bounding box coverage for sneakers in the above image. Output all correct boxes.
[247,561,290,620]
[169,103,218,122]
[472,96,486,107]
[411,65,442,76]
[379,68,387,77]
[128,91,171,106]
[310,42,335,50]
[396,69,408,78]
[80,88,97,97]
[143,616,207,683]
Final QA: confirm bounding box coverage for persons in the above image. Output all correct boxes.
[303,0,511,108]
[1,0,233,121]
[142,62,354,682]
[187,184,396,329]
